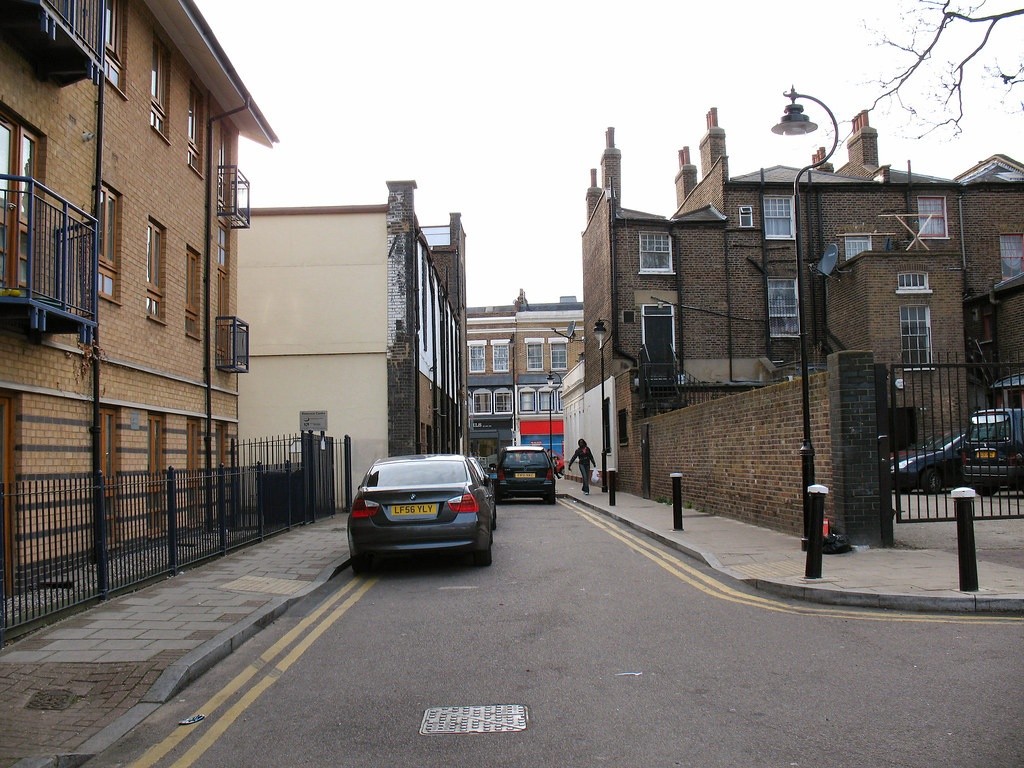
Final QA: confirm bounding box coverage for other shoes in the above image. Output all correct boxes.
[558,477,562,479]
[582,490,589,495]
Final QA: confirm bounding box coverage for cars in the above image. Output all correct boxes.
[347,453,496,573]
[890,408,1024,497]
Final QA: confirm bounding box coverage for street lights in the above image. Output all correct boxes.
[547,370,561,460]
[593,320,613,493]
[771,82,838,551]
[508,332,517,446]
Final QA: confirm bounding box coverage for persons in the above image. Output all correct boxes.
[553,456,559,467]
[547,449,565,479]
[568,439,596,495]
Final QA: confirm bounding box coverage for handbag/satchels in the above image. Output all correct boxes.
[591,465,602,484]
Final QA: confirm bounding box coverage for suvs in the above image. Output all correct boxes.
[489,445,564,504]
